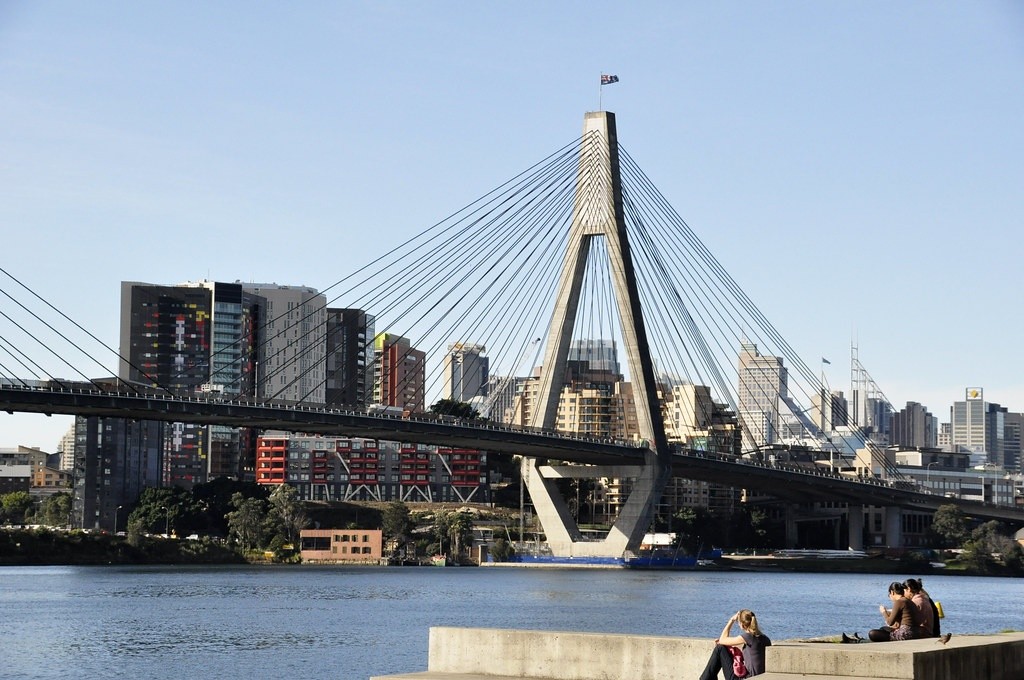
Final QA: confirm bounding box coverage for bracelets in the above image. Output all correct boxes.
[731,619,734,623]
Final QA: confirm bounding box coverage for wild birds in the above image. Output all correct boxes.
[934,633,952,645]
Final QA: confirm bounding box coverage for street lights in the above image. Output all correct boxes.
[927,461,940,489]
[161,506,169,534]
[114,505,122,534]
[985,462,998,504]
[254,361,259,407]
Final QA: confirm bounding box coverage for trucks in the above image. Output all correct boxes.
[944,491,957,498]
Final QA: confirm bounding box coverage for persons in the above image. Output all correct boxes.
[901,579,934,638]
[699,608,772,680]
[917,578,940,637]
[868,582,920,642]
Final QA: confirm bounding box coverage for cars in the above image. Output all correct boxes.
[185,533,200,540]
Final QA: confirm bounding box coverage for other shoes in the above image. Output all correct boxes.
[842,633,851,642]
[854,632,861,642]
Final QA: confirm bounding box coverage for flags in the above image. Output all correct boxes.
[601,74,619,85]
[823,357,830,364]
